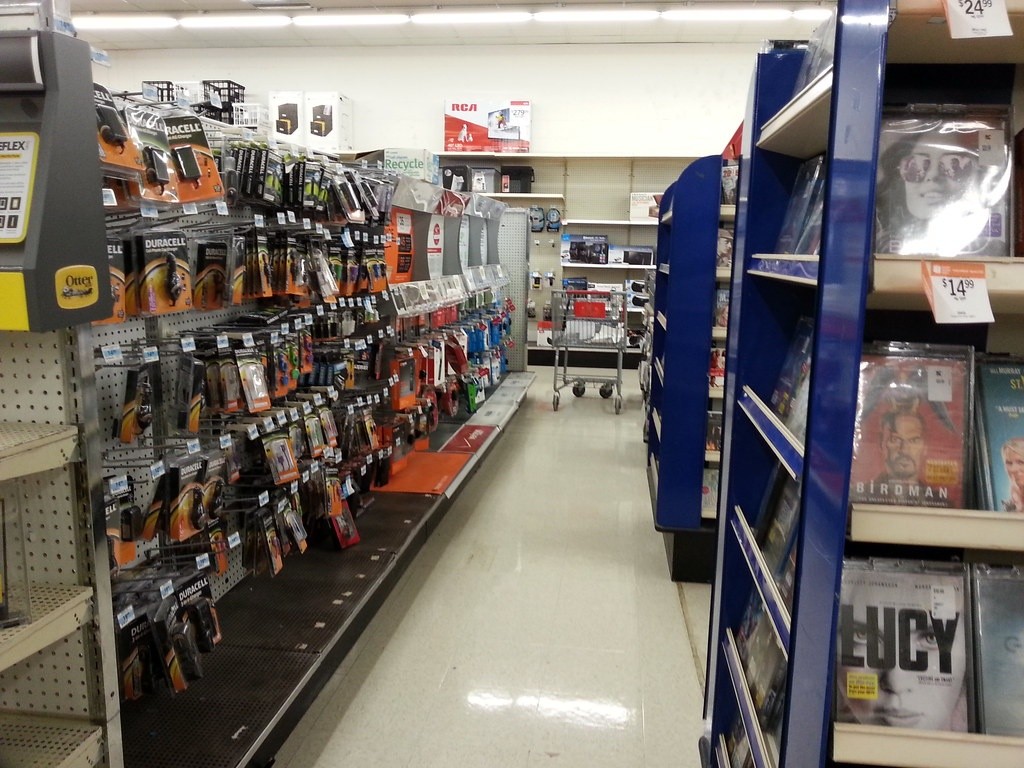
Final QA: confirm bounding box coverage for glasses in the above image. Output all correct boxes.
[896,150,979,183]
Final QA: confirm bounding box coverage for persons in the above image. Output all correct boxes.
[1000,438,1024,513]
[838,570,967,733]
[855,409,954,509]
[875,124,1005,257]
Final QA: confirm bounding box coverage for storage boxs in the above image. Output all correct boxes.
[304,90,355,154]
[234,103,259,131]
[268,91,306,152]
[201,79,246,111]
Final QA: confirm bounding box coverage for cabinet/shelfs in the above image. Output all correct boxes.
[0,0,1024,768]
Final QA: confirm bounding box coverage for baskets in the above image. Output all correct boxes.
[143,79,268,139]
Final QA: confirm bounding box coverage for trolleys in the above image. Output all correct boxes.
[552,289,628,416]
